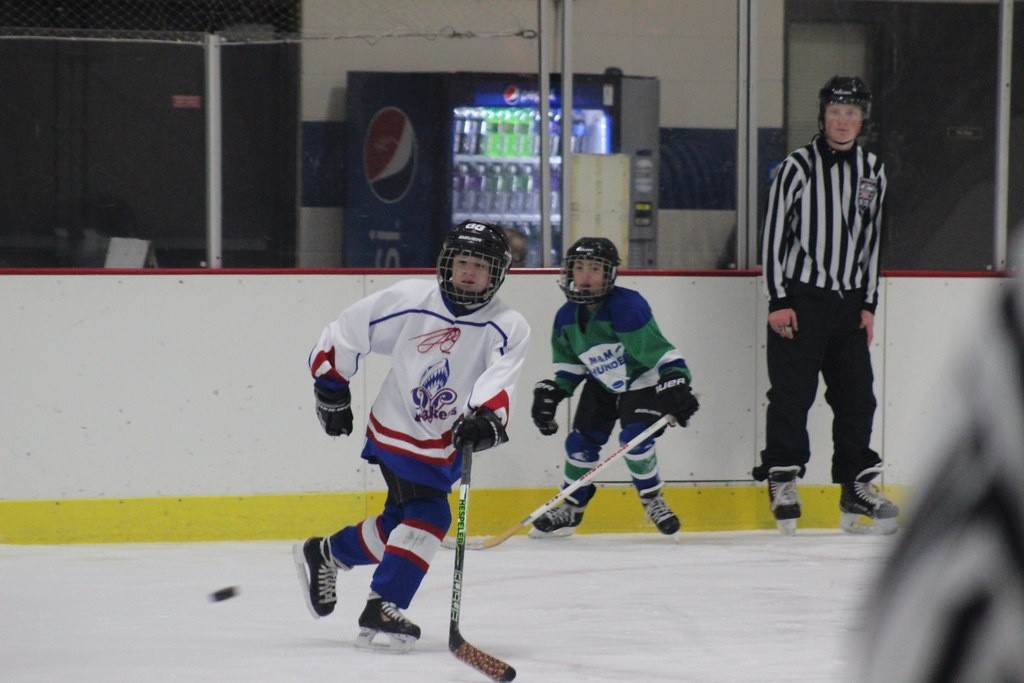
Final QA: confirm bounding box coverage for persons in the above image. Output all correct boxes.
[529,237,700,540]
[751,74,901,535]
[291,219,531,654]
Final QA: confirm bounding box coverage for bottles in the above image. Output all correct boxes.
[450,107,592,268]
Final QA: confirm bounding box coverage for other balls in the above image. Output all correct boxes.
[211,587,235,602]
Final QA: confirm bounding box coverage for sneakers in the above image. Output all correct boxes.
[639,481,681,543]
[293,537,340,619]
[352,598,421,653]
[767,465,801,537]
[838,463,899,536]
[528,484,597,539]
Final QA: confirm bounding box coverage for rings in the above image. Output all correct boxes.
[778,327,784,333]
[784,325,793,337]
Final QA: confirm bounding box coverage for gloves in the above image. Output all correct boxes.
[314,382,353,436]
[531,379,571,435]
[452,407,509,453]
[655,371,700,427]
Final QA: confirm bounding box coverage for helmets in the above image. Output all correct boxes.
[557,237,622,305]
[818,75,873,137]
[436,219,513,306]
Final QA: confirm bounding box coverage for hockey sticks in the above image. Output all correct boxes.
[446,414,518,683]
[441,390,701,552]
